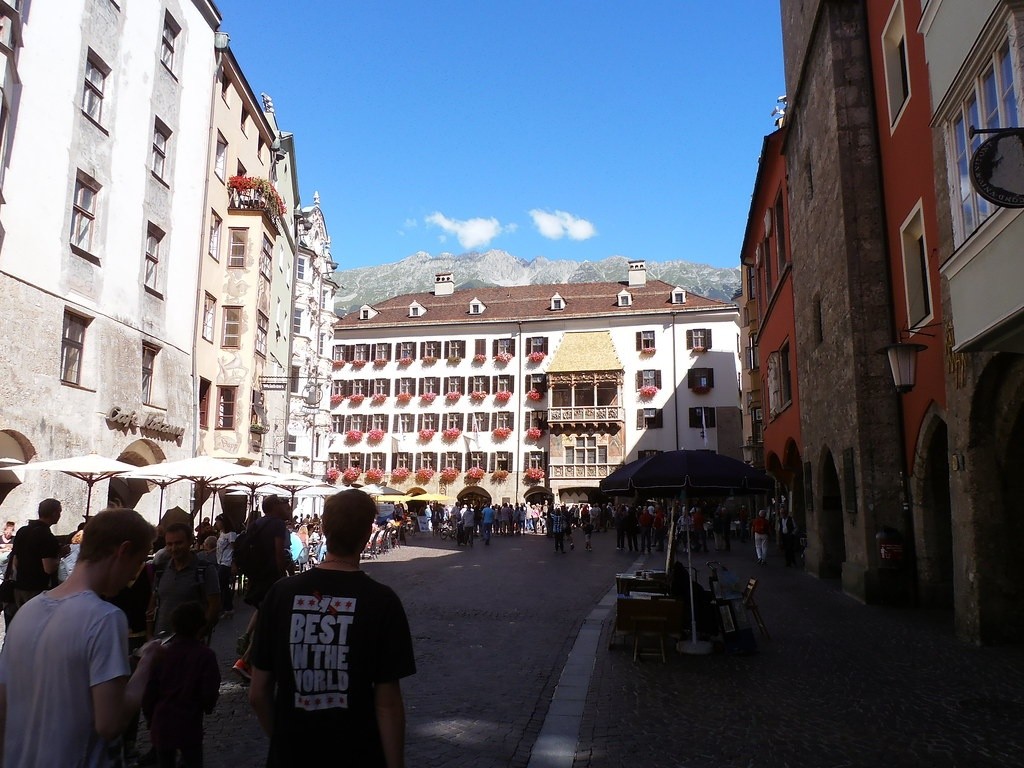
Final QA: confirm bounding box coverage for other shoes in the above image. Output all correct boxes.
[486,539,489,545]
[616,546,625,550]
[570,543,575,550]
[757,559,765,566]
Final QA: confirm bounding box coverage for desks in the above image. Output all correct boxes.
[615,572,676,594]
[614,592,708,662]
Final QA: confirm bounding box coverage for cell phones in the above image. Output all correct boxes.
[132,631,176,658]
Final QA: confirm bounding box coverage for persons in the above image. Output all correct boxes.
[135,603,222,766]
[0,479,813,680]
[6,508,178,767]
[245,490,418,767]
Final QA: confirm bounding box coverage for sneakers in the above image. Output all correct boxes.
[234,659,253,682]
[236,634,250,657]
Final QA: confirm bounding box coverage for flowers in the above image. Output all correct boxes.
[496,350,514,364]
[492,426,512,444]
[445,391,462,400]
[640,385,658,397]
[474,354,486,363]
[325,355,438,483]
[228,174,287,219]
[440,464,460,482]
[448,354,462,364]
[472,390,488,399]
[465,467,485,480]
[693,386,711,394]
[692,345,707,353]
[493,465,510,481]
[442,427,461,440]
[526,466,545,480]
[529,352,545,362]
[527,388,545,403]
[528,428,546,440]
[642,347,656,354]
[495,390,512,402]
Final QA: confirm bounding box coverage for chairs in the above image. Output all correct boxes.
[238,190,253,209]
[729,577,769,634]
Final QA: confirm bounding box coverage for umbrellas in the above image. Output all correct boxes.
[0,446,457,550]
[598,448,788,507]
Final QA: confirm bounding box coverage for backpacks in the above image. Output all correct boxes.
[233,517,279,575]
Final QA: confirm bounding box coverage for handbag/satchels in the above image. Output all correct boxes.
[0,573,15,602]
[636,521,642,535]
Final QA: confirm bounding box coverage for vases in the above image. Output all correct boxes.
[695,347,704,351]
[646,348,651,352]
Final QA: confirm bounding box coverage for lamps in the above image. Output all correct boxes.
[875,320,942,394]
